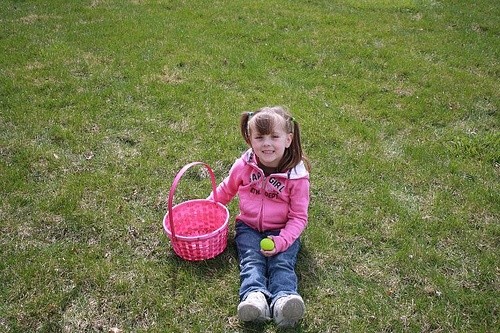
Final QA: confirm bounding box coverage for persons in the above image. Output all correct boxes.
[200,106,311,328]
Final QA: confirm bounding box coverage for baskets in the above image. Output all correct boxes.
[163,161,229,261]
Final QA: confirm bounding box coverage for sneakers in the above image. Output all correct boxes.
[237,291,270,321]
[273,295,305,326]
